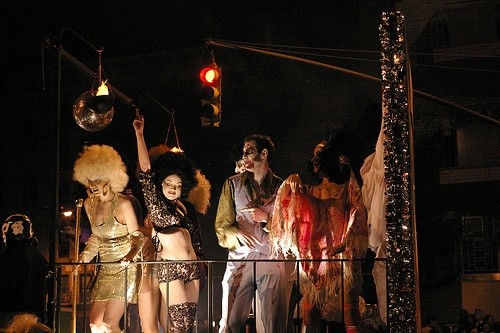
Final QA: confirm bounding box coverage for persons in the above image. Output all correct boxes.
[66,146,146,333]
[134,116,211,333]
[0,214,50,333]
[215,133,370,333]
[421,308,500,333]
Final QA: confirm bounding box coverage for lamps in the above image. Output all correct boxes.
[199,49,222,127]
